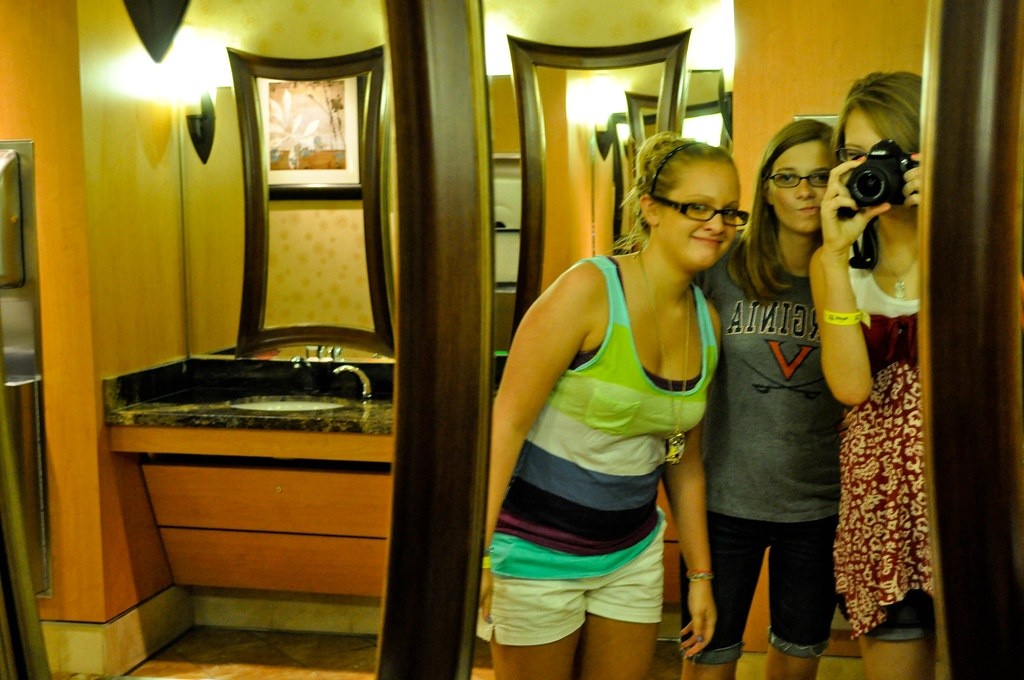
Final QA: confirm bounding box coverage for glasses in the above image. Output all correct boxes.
[769,171,830,188]
[835,144,862,162]
[651,194,749,226]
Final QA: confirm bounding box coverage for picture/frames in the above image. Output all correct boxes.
[255,75,368,199]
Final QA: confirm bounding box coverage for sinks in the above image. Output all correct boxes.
[227,399,347,412]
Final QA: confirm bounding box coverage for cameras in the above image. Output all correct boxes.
[846,139,918,206]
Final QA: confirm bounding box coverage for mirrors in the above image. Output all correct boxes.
[457,0,1024,680]
[224,46,393,359]
[505,27,724,345]
[0,0,392,680]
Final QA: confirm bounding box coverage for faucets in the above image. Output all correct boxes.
[318,345,335,360]
[333,365,373,401]
[290,355,318,394]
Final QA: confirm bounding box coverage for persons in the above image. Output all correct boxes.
[678,119,844,680]
[805,71,942,680]
[477,131,750,680]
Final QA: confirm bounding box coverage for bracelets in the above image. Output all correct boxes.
[483,548,489,552]
[482,557,491,568]
[823,310,871,330]
[686,569,714,582]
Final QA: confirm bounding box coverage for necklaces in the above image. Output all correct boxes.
[879,245,918,298]
[638,252,690,464]
